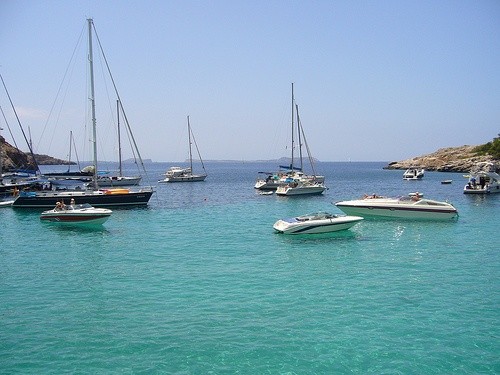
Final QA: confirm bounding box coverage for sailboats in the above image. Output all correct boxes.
[159,115,208,183]
[83,99,142,187]
[0,74,83,194]
[255,81,327,196]
[11,17,155,208]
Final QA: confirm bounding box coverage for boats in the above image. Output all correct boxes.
[273,212,363,235]
[403,167,424,180]
[464,170,500,194]
[40,203,114,225]
[162,166,191,176]
[335,197,457,221]
